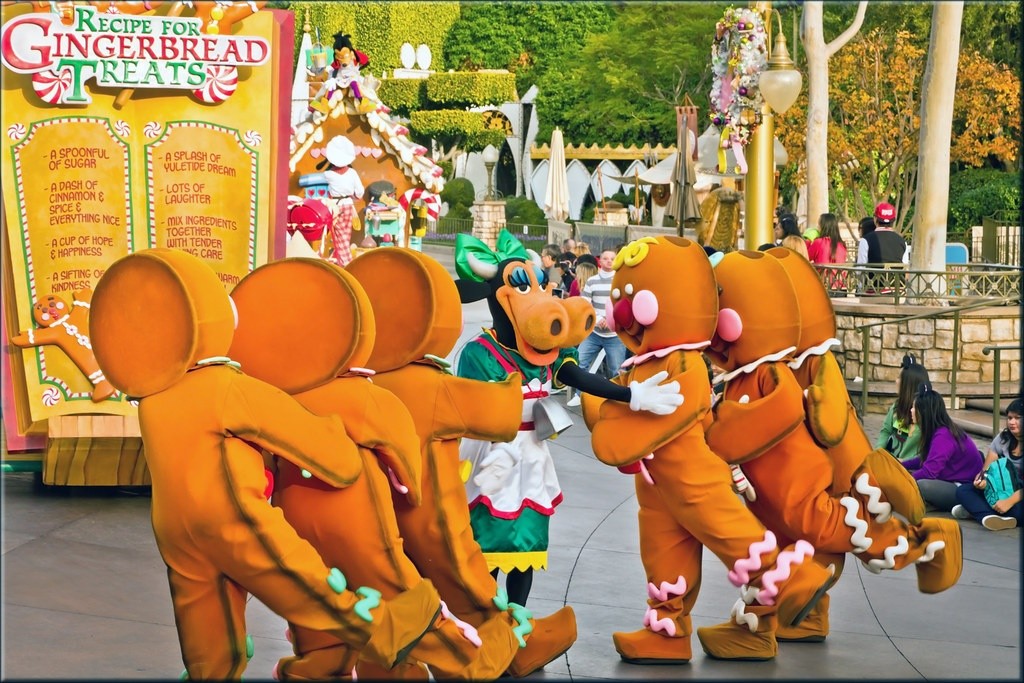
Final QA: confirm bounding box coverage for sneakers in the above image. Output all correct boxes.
[566,395,581,407]
[550,386,568,394]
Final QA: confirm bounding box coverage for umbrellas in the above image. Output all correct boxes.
[544,125,570,222]
[664,127,702,237]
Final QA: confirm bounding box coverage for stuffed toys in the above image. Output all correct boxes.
[226,258,537,683]
[581,236,836,664]
[310,31,375,114]
[454,230,683,607]
[345,247,577,680]
[696,249,925,660]
[764,245,963,644]
[316,135,364,265]
[88,247,442,683]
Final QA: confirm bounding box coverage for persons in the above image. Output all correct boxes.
[542,240,635,407]
[776,213,847,297]
[853,203,908,297]
[901,390,983,512]
[951,398,1024,530]
[873,354,932,462]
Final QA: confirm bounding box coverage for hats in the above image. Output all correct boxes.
[873,203,896,222]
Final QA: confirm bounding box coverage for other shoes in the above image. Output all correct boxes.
[952,505,971,520]
[982,514,1016,530]
